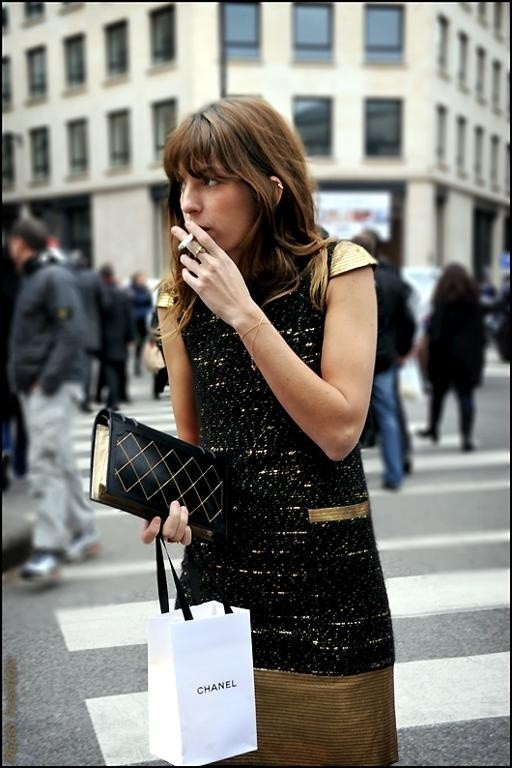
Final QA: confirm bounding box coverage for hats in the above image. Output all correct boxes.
[12,216,49,247]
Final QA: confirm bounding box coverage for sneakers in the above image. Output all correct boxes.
[17,528,100,589]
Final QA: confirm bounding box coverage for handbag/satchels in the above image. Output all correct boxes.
[90,408,223,545]
[148,530,258,768]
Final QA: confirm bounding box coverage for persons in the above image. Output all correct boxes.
[139,101,401,768]
[3,219,105,581]
